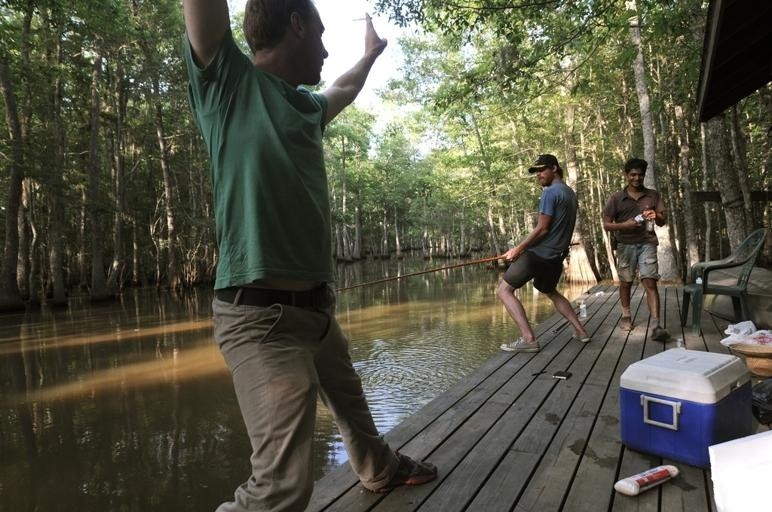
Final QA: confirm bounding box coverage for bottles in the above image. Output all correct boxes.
[647,214,654,231]
[633,213,648,224]
[579,300,588,317]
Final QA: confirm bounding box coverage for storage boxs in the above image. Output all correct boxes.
[619,347,753,469]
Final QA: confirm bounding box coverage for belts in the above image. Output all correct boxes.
[214,283,336,311]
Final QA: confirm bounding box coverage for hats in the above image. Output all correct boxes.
[728,341,772,378]
[363,449,439,494]
[528,154,558,173]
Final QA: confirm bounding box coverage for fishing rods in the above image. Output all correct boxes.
[335,244,581,292]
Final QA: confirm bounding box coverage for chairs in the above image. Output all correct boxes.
[680,228,770,337]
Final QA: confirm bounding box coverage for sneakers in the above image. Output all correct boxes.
[650,325,671,340]
[572,330,590,343]
[500,337,539,353]
[618,316,633,331]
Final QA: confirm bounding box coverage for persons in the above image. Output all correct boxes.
[176,0,440,510]
[602,157,672,341]
[494,154,593,353]
